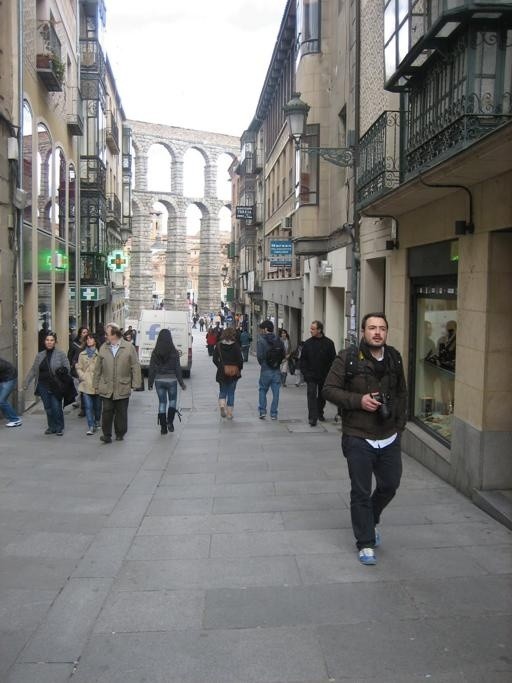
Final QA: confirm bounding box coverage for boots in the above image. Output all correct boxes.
[228,406,233,419]
[218,399,226,417]
[158,413,167,434]
[167,407,176,432]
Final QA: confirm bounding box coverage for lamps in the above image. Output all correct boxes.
[221,264,243,287]
[455,220,475,236]
[279,93,357,169]
[385,239,400,252]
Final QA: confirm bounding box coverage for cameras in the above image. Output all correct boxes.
[373,391,393,421]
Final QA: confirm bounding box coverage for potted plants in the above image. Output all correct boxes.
[37,50,66,88]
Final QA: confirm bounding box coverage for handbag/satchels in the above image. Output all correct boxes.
[224,365,241,377]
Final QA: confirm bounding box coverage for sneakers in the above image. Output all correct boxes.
[260,412,277,420]
[6,420,22,427]
[359,525,380,565]
[309,415,325,426]
[72,401,124,443]
[45,428,64,436]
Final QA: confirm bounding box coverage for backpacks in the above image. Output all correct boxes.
[263,338,284,369]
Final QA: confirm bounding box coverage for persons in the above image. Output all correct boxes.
[213,327,243,418]
[68,323,136,436]
[38,322,51,350]
[422,319,456,417]
[149,329,186,434]
[322,312,409,568]
[23,332,74,438]
[301,319,336,426]
[1,358,21,427]
[192,311,307,388]
[256,320,285,420]
[93,321,142,443]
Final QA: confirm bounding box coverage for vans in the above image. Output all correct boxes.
[135,308,194,379]
